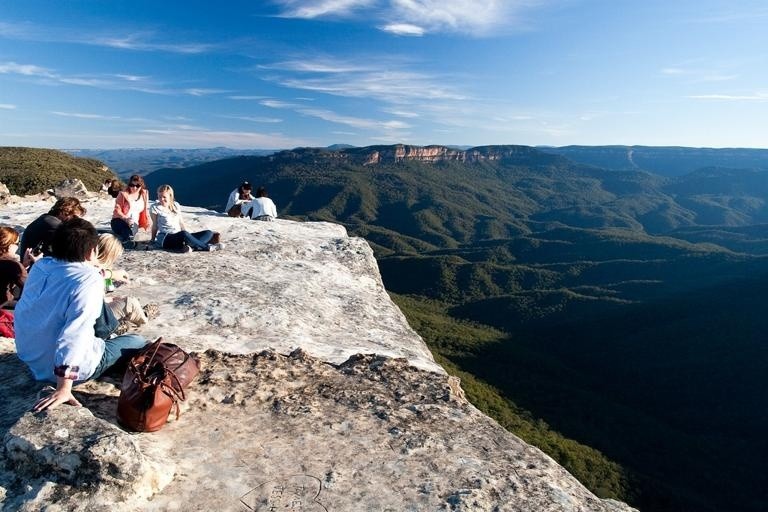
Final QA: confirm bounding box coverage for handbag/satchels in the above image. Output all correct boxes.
[116,336,201,433]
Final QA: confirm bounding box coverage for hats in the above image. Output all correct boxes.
[242,181,252,190]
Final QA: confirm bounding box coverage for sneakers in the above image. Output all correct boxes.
[183,245,192,253]
[207,243,225,253]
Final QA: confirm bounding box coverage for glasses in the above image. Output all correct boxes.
[128,183,141,188]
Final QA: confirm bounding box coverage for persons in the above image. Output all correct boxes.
[225,180,255,218]
[94,228,159,340]
[101,179,112,191]
[107,180,121,198]
[148,184,226,252]
[20,197,87,275]
[13,216,155,412]
[1,225,45,310]
[241,186,278,223]
[111,175,150,249]
